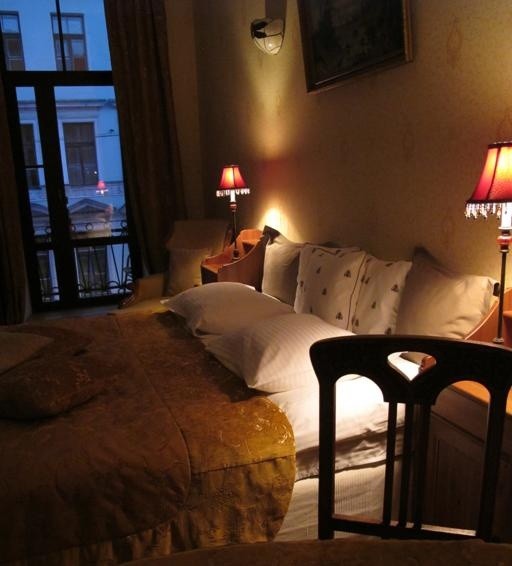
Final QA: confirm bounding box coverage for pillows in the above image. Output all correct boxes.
[0,324,130,421]
[160,281,297,339]
[164,242,213,297]
[262,240,499,362]
[205,314,357,394]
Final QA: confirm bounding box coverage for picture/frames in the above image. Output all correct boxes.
[296,0,414,94]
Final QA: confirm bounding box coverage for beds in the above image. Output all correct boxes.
[0,311,512,566]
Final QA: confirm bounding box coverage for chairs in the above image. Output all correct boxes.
[119,219,234,309]
[309,333,512,542]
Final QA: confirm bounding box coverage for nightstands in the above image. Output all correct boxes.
[200,225,285,283]
[408,287,512,541]
[414,372,512,530]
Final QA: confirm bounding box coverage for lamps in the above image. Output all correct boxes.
[216,163,251,260]
[250,16,285,56]
[463,141,512,345]
[95,179,109,196]
